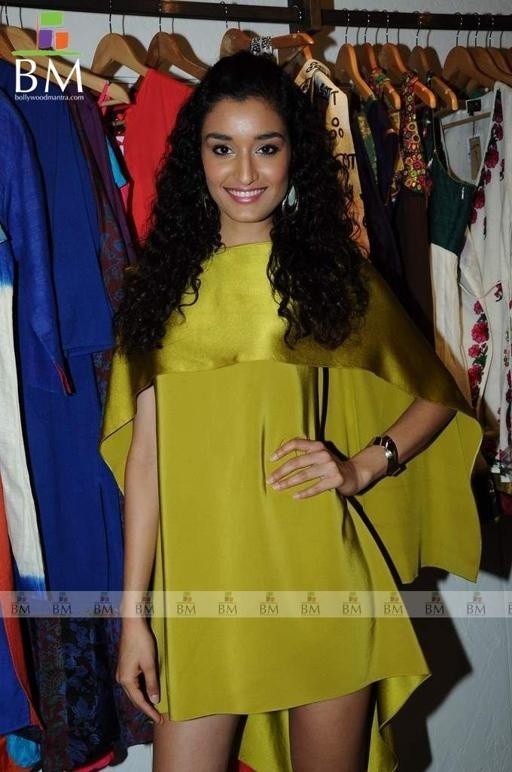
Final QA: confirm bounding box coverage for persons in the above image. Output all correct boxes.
[99,54,486,772]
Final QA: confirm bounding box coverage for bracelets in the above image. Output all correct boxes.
[370,434,405,479]
